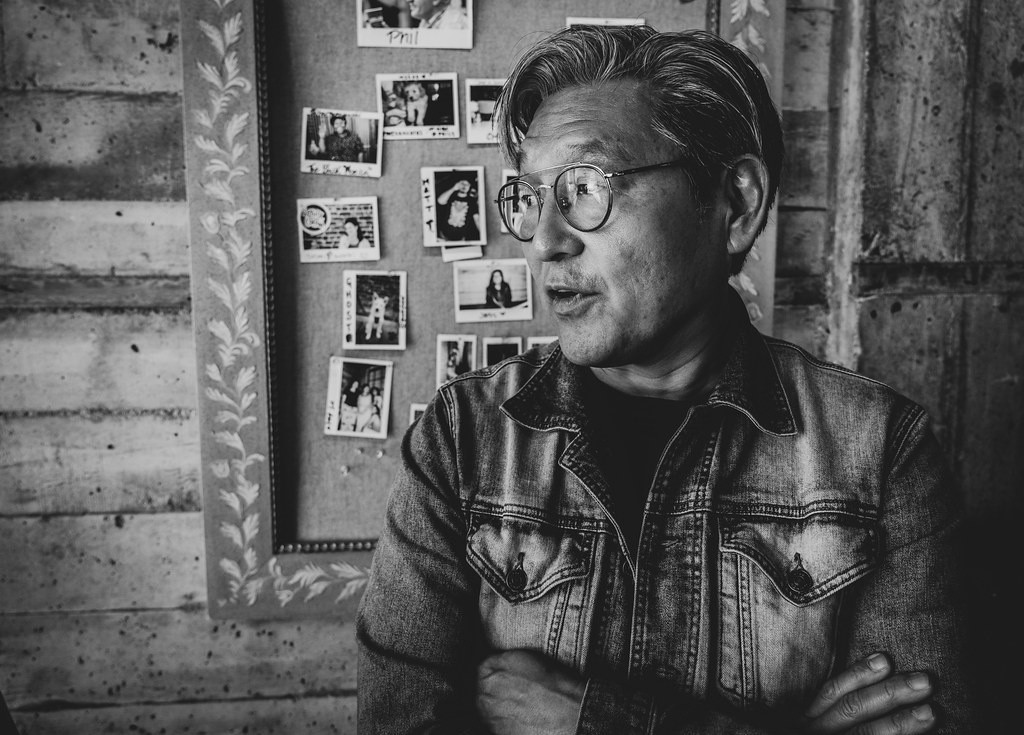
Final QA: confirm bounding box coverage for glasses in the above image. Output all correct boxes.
[493,159,725,242]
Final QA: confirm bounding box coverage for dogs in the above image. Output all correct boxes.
[363,292,389,339]
[404,82,428,126]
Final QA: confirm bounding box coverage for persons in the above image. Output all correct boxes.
[324,113,365,163]
[486,270,512,308]
[340,381,382,433]
[437,175,481,241]
[339,217,371,248]
[353,26,974,735]
[361,0,468,29]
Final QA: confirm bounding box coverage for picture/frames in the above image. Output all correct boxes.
[178,0,784,628]
